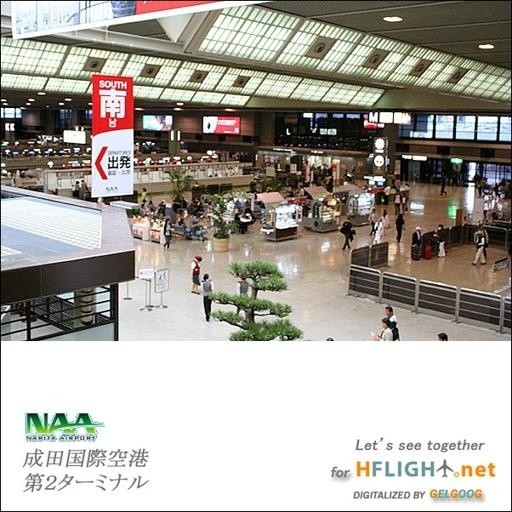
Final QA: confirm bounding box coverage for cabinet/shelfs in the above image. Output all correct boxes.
[259,193,377,242]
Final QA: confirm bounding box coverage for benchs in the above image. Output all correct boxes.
[172,224,190,241]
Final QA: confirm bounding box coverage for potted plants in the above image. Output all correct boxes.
[203,190,239,252]
[161,165,196,220]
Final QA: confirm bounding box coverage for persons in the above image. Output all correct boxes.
[155,115,172,131]
[236,276,250,315]
[75,181,80,191]
[384,306,400,341]
[141,188,216,243]
[191,256,203,294]
[437,333,447,341]
[339,165,500,265]
[201,273,214,322]
[372,318,394,341]
[81,180,87,192]
[163,218,174,251]
